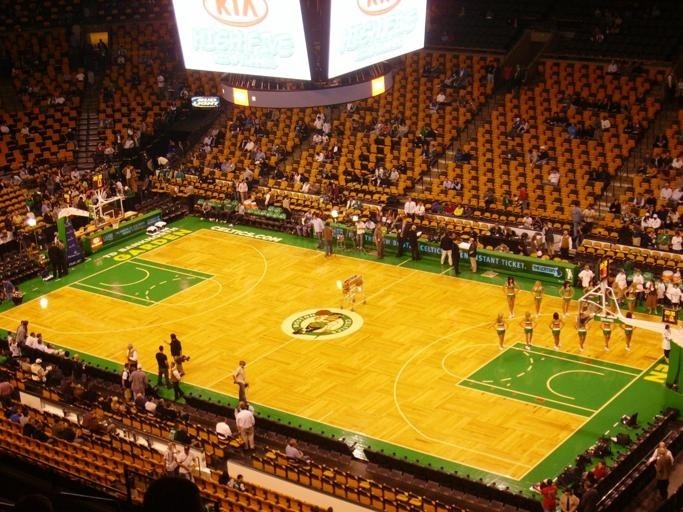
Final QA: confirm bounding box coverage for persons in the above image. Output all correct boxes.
[486,312,510,351]
[598,311,616,353]
[504,276,519,315]
[573,311,592,352]
[545,57,681,186]
[558,282,573,312]
[285,439,307,468]
[1,159,135,300]
[144,433,204,512]
[0,320,121,443]
[471,2,661,44]
[532,280,544,311]
[619,311,638,353]
[572,180,683,314]
[662,324,671,366]
[517,310,538,351]
[144,55,189,118]
[313,100,438,187]
[420,27,528,111]
[121,334,184,420]
[190,109,332,226]
[310,185,478,277]
[547,311,566,351]
[507,116,549,165]
[218,470,246,492]
[95,124,184,191]
[2,3,177,146]
[525,410,683,512]
[216,362,255,452]
[442,148,471,191]
[483,188,572,259]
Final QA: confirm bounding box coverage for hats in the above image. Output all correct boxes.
[645,212,650,217]
[36,358,42,364]
[653,213,658,218]
